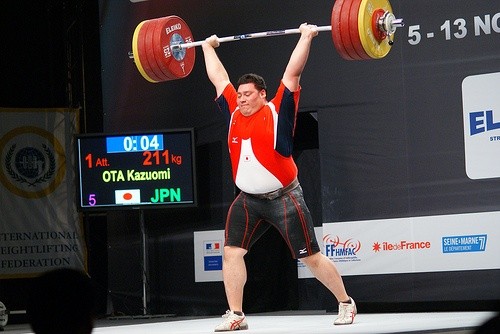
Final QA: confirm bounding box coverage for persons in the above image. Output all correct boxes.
[201,21,357,331]
[25,268,97,334]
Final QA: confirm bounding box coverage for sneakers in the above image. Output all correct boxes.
[333,297,357,325]
[214,311,248,332]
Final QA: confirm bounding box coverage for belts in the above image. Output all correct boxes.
[243,176,301,200]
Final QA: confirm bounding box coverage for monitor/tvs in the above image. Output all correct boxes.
[73,127,198,213]
